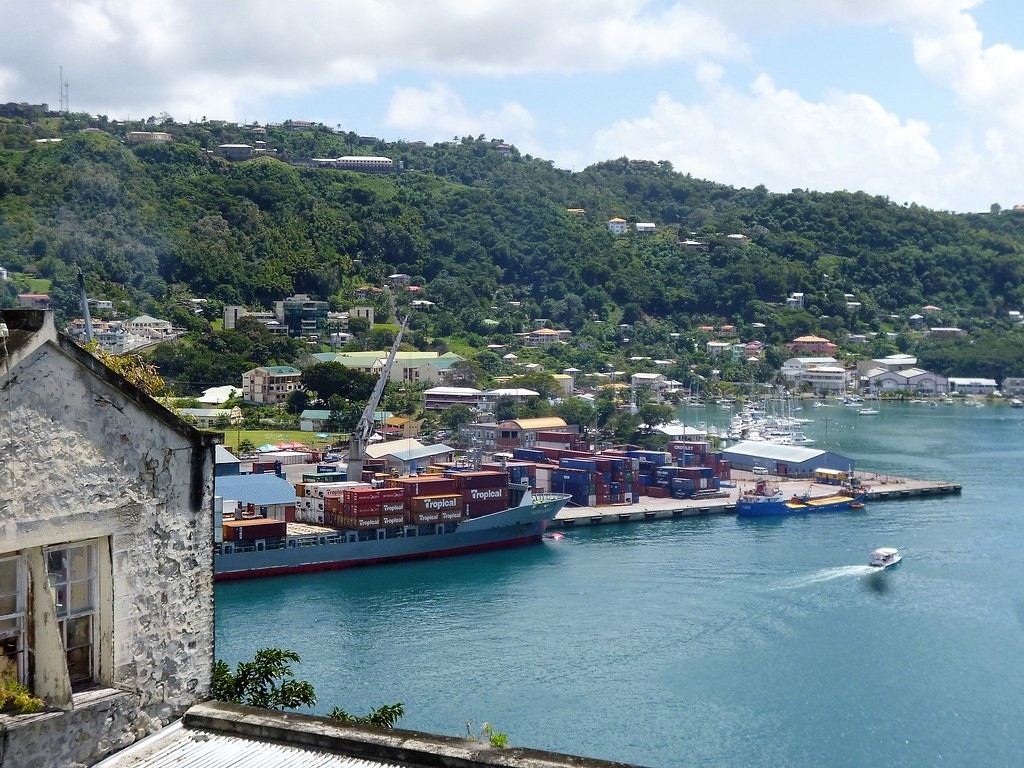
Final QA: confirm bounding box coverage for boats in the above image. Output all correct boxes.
[964,395,979,406]
[1010,399,1023,407]
[735,464,872,517]
[727,373,815,446]
[716,397,734,410]
[844,398,864,407]
[870,548,903,569]
[214,486,573,580]
[813,400,821,408]
[929,400,937,408]
[942,398,953,405]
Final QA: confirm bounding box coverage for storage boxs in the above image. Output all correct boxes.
[222,430,730,543]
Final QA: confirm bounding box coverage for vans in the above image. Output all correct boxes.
[753,467,768,475]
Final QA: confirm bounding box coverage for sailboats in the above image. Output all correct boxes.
[682,380,705,408]
[857,379,880,416]
[835,372,865,402]
